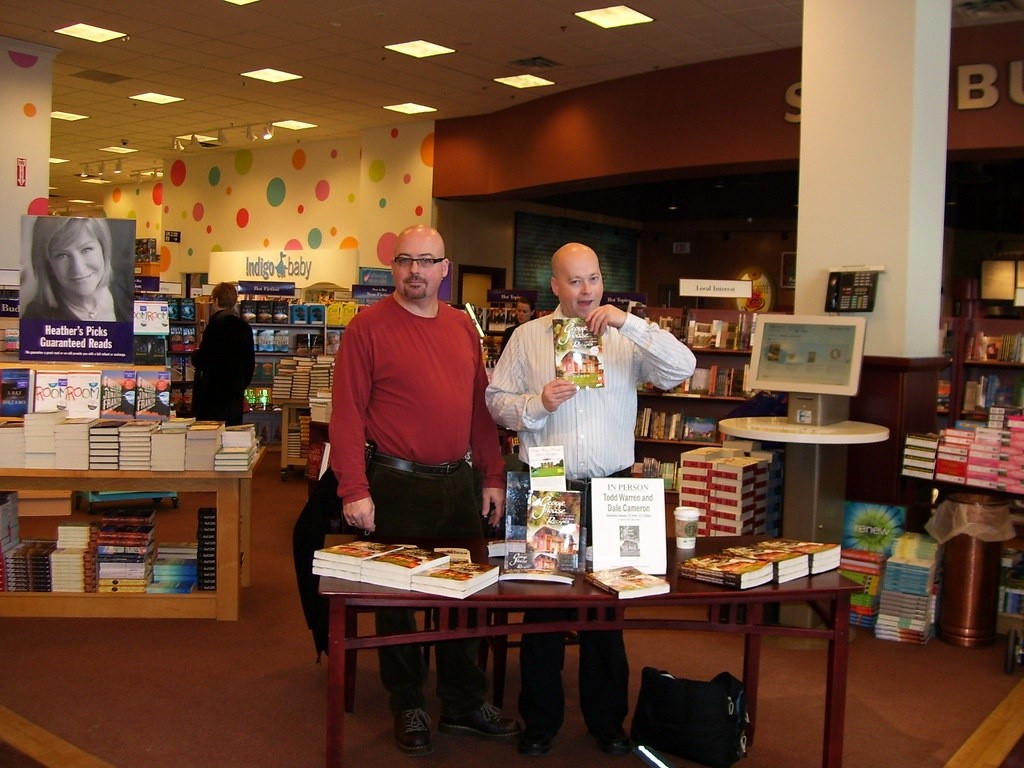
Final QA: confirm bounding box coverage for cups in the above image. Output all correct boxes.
[673,506,700,549]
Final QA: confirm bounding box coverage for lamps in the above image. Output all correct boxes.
[153,168,157,180]
[247,126,257,145]
[269,123,274,137]
[114,163,118,174]
[217,130,229,145]
[136,175,144,183]
[134,171,141,184]
[172,136,177,150]
[81,163,88,177]
[262,122,273,140]
[117,159,122,173]
[177,140,184,152]
[151,173,156,181]
[191,134,202,152]
[98,161,104,175]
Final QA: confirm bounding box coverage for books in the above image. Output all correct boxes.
[551,318,606,389]
[85,507,217,594]
[488,445,671,598]
[0,368,172,421]
[0,418,195,471]
[292,305,324,324]
[325,303,357,327]
[288,387,333,459]
[239,300,288,411]
[135,296,197,417]
[304,440,331,481]
[297,333,323,355]
[632,312,757,489]
[186,420,263,471]
[272,356,335,399]
[312,539,500,599]
[327,330,345,355]
[0,490,100,594]
[257,327,288,381]
[677,537,841,589]
[839,276,1024,646]
[679,440,784,537]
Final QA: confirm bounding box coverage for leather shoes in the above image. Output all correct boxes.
[437,700,521,740]
[394,707,433,757]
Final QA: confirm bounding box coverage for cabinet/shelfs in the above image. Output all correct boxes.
[0,249,1024,650]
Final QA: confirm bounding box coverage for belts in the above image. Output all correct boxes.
[371,452,462,474]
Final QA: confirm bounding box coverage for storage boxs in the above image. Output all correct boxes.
[682,467,707,476]
[708,524,752,534]
[698,515,710,522]
[135,238,161,262]
[706,469,755,480]
[710,490,755,500]
[679,492,708,502]
[711,503,757,513]
[698,508,709,516]
[712,477,756,487]
[683,458,729,469]
[683,479,707,489]
[697,521,708,529]
[708,483,752,493]
[681,448,720,461]
[681,487,710,496]
[679,500,711,510]
[709,496,754,507]
[707,510,752,521]
[683,474,712,482]
[711,517,755,528]
[717,457,759,473]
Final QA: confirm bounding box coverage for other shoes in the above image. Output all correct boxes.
[517,728,557,755]
[590,724,630,758]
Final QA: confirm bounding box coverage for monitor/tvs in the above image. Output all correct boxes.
[747,314,866,396]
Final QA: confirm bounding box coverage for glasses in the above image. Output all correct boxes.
[392,256,446,268]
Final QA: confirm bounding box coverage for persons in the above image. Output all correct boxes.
[485,242,697,756]
[21,215,134,321]
[328,223,522,755]
[500,297,539,359]
[190,282,255,425]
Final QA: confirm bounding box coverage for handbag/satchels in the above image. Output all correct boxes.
[628,666,749,768]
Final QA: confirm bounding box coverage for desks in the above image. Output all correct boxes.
[318,533,866,768]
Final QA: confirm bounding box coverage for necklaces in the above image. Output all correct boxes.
[60,290,99,319]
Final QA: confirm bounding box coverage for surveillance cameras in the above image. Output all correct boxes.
[120,138,128,145]
